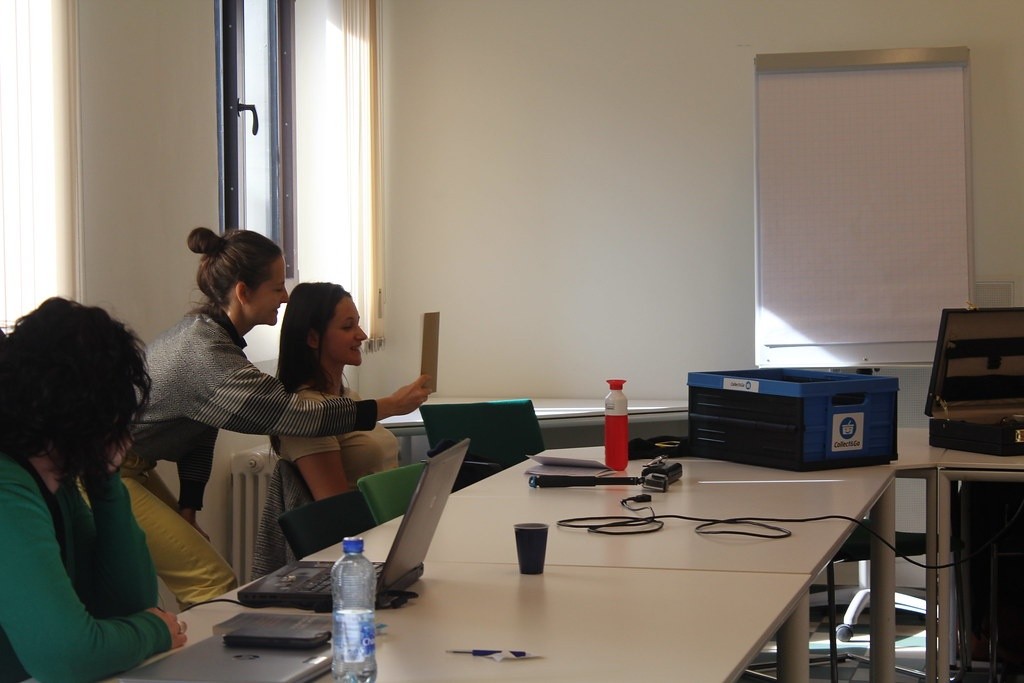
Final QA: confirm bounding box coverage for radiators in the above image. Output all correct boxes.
[230,447,280,590]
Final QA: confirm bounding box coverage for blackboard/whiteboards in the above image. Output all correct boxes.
[746,40,979,373]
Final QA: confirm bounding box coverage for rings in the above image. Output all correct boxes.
[176,620,184,635]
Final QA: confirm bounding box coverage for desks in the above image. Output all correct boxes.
[21,424,1024,683]
[366,399,686,468]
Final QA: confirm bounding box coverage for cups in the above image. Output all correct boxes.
[514,523,549,575]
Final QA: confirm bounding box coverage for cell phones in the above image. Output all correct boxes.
[223,626,330,649]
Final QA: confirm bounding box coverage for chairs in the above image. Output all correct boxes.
[274,400,548,561]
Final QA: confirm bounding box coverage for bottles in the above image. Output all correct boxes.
[604,380,628,471]
[330,537,377,683]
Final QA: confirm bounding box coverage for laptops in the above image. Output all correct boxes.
[118,636,334,683]
[237,438,472,610]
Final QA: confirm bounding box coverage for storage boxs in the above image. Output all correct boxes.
[687,370,900,472]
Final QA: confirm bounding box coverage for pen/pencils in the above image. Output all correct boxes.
[445,649,541,661]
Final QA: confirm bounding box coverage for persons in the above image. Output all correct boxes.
[0,297,188,683]
[270,280,400,502]
[76,227,432,612]
